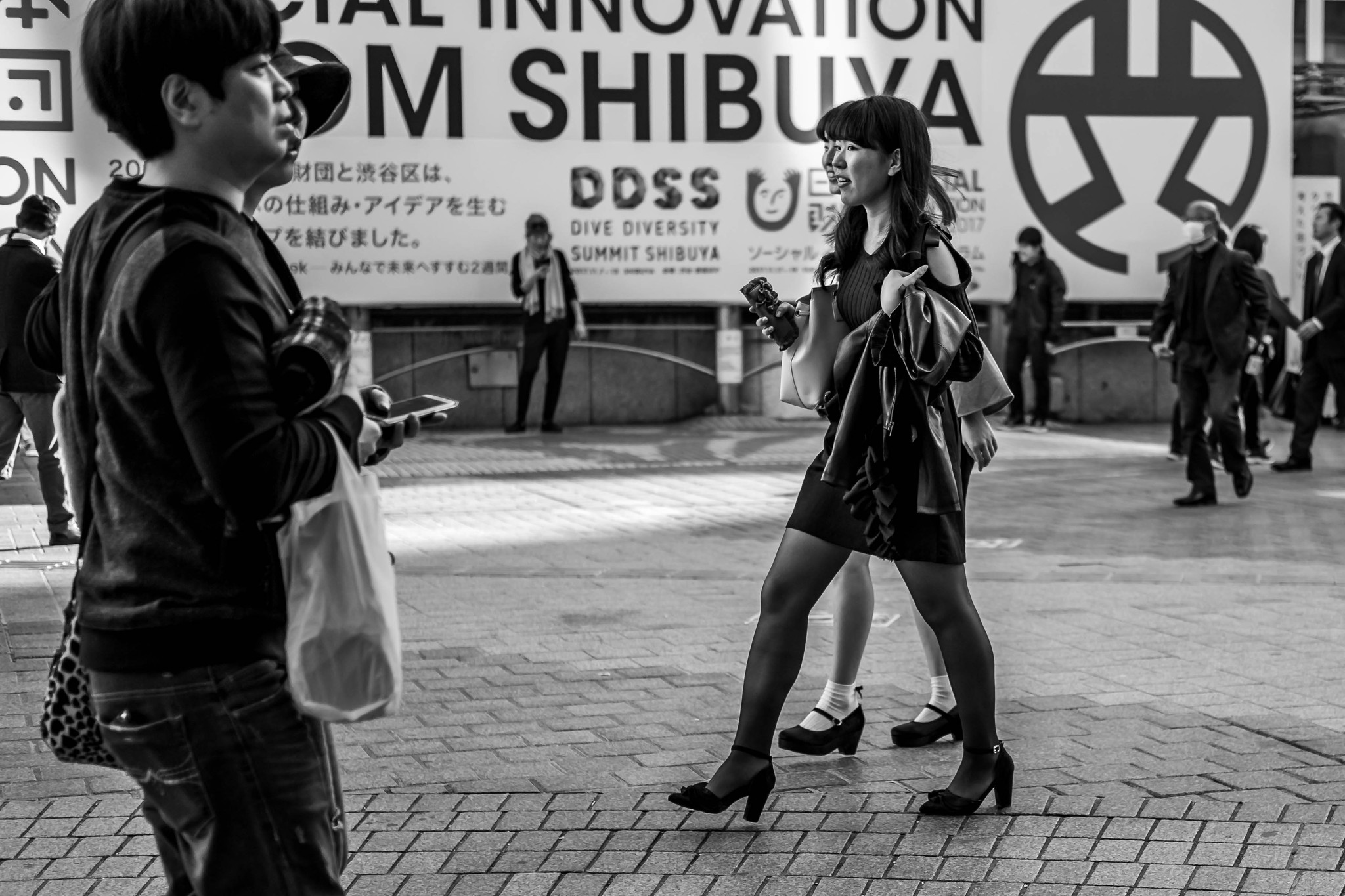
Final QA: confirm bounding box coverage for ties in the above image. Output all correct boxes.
[1315,252,1323,304]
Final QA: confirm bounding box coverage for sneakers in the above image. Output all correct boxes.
[1232,464,1253,499]
[1173,483,1217,506]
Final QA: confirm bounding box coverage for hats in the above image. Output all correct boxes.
[269,43,351,140]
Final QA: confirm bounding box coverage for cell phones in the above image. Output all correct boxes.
[364,393,459,426]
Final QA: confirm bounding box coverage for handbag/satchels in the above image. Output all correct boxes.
[39,597,124,770]
[1270,367,1305,421]
[779,270,852,410]
[277,418,403,723]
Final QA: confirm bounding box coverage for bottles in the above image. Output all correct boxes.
[1244,334,1272,376]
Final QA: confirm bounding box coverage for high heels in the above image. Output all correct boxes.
[891,702,964,747]
[668,745,776,823]
[920,739,1015,818]
[778,703,865,756]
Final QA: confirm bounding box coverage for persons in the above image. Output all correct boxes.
[1150,200,1271,506]
[59,0,449,895]
[997,227,1067,433]
[502,215,587,433]
[1166,224,1303,470]
[668,96,1015,823]
[1273,202,1345,470]
[0,195,80,545]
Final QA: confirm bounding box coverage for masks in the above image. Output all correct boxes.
[1184,221,1214,245]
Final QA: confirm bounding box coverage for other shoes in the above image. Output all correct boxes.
[1271,456,1313,473]
[995,417,1027,431]
[504,423,527,434]
[1167,435,1275,470]
[1021,419,1050,433]
[541,423,563,434]
[49,526,81,546]
